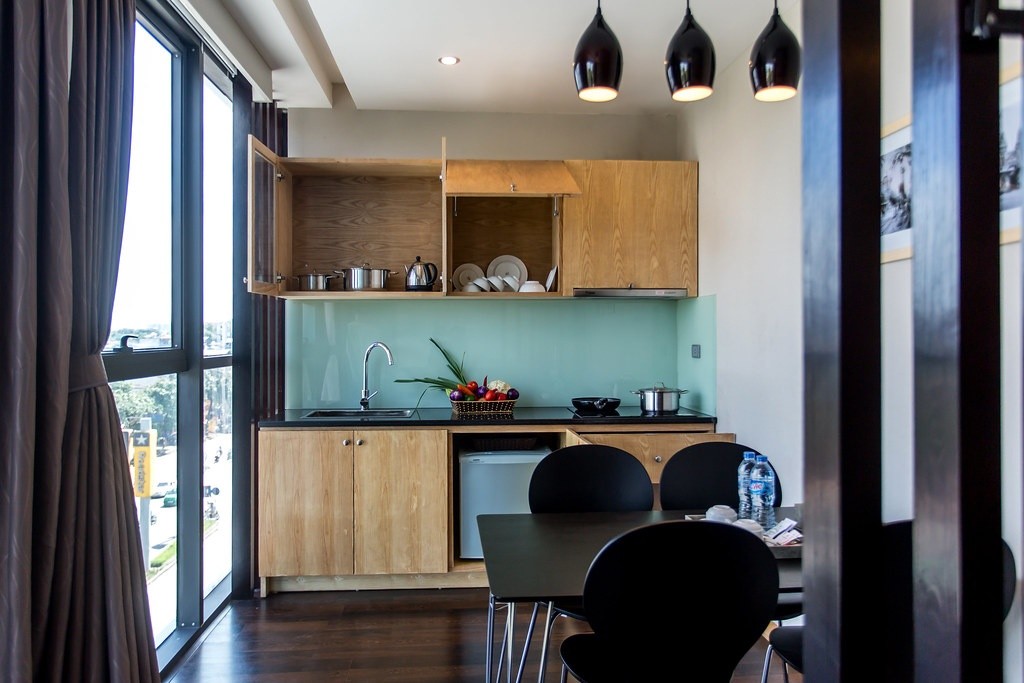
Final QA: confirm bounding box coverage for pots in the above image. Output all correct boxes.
[334,263,399,291]
[572,398,621,412]
[630,381,689,415]
[291,268,339,291]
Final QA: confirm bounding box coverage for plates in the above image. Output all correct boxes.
[546,265,557,291]
[453,263,485,288]
[486,255,528,292]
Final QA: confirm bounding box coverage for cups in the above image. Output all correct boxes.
[705,505,737,523]
[732,519,764,541]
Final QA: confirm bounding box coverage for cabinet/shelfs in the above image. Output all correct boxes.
[258,430,450,578]
[244,134,699,298]
[578,432,738,509]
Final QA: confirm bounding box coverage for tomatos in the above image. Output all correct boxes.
[485,390,508,400]
[467,381,478,391]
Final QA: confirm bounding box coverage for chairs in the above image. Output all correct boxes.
[560,520,779,683]
[761,521,1016,683]
[659,442,805,628]
[516,444,653,683]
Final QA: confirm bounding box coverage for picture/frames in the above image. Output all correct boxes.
[881,61,1023,263]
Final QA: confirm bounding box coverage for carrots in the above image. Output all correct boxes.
[457,384,478,400]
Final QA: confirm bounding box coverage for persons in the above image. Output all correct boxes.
[205,503,215,517]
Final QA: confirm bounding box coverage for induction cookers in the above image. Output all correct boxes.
[567,406,696,417]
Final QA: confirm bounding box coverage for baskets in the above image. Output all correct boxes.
[451,411,514,422]
[450,399,518,414]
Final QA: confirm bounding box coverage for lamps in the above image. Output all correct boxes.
[664,0,716,102]
[749,0,800,102]
[571,0,623,102]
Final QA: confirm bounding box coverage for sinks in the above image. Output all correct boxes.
[299,408,416,418]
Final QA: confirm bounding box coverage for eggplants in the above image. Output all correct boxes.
[450,390,465,401]
[507,388,519,400]
[476,385,489,397]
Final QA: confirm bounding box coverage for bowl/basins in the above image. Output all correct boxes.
[487,275,505,292]
[461,281,481,292]
[518,281,546,292]
[502,275,520,292]
[473,277,490,292]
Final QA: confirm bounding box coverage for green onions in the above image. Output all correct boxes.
[393,338,467,408]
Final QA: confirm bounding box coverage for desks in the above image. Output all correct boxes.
[476,506,805,683]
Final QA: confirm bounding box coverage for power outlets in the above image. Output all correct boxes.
[692,344,700,357]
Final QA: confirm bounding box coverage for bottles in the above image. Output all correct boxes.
[750,455,775,529]
[738,451,757,518]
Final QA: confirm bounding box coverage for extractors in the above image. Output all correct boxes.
[573,287,688,298]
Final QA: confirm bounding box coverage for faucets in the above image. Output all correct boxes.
[359,341,394,410]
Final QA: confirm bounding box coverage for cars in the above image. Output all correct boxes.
[150,481,177,508]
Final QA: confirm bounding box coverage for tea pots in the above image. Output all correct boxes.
[403,255,438,291]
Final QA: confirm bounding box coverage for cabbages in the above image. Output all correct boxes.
[489,380,511,394]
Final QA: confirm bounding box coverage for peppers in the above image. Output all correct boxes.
[483,376,488,387]
